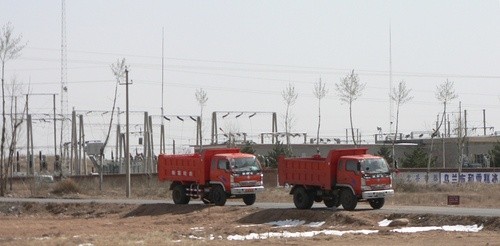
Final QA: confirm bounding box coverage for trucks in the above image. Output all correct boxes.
[278,148,395,211]
[157,148,264,206]
